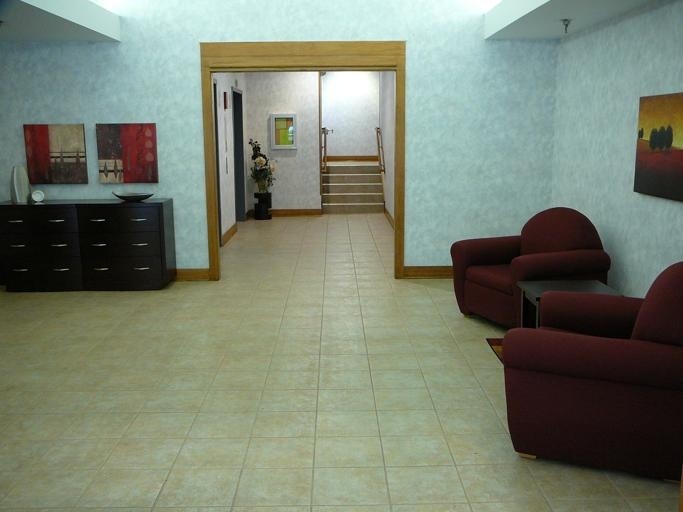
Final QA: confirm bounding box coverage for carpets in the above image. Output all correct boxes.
[485,337,503,365]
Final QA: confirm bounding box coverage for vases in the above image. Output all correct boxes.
[253,192,272,220]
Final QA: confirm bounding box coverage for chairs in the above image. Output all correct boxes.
[501,261,683,483]
[450,206,612,328]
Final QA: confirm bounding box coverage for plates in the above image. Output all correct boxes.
[31,189,44,201]
[111,190,154,203]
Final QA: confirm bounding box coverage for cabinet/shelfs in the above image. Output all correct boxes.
[0,196,177,292]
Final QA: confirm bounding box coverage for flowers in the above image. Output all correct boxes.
[248,138,276,193]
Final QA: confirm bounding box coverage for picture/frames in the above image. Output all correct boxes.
[270,113,298,150]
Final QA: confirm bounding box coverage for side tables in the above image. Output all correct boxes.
[514,279,624,329]
